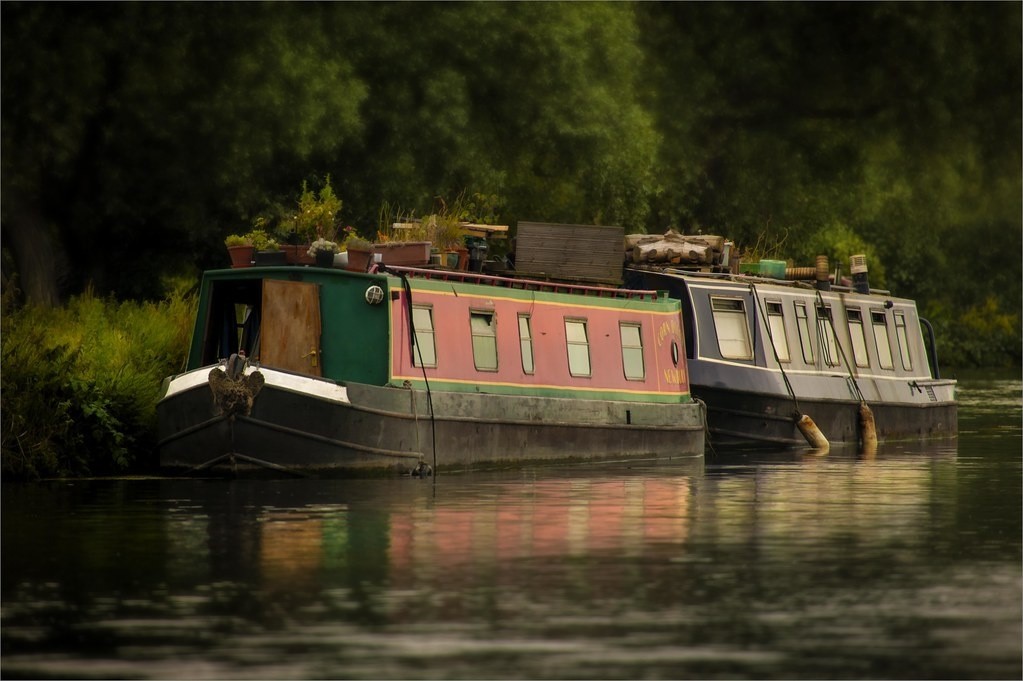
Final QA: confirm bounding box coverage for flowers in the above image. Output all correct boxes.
[343,226,367,243]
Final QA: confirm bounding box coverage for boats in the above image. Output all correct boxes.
[146,254,958,480]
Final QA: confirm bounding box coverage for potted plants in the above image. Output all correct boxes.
[223,174,487,273]
